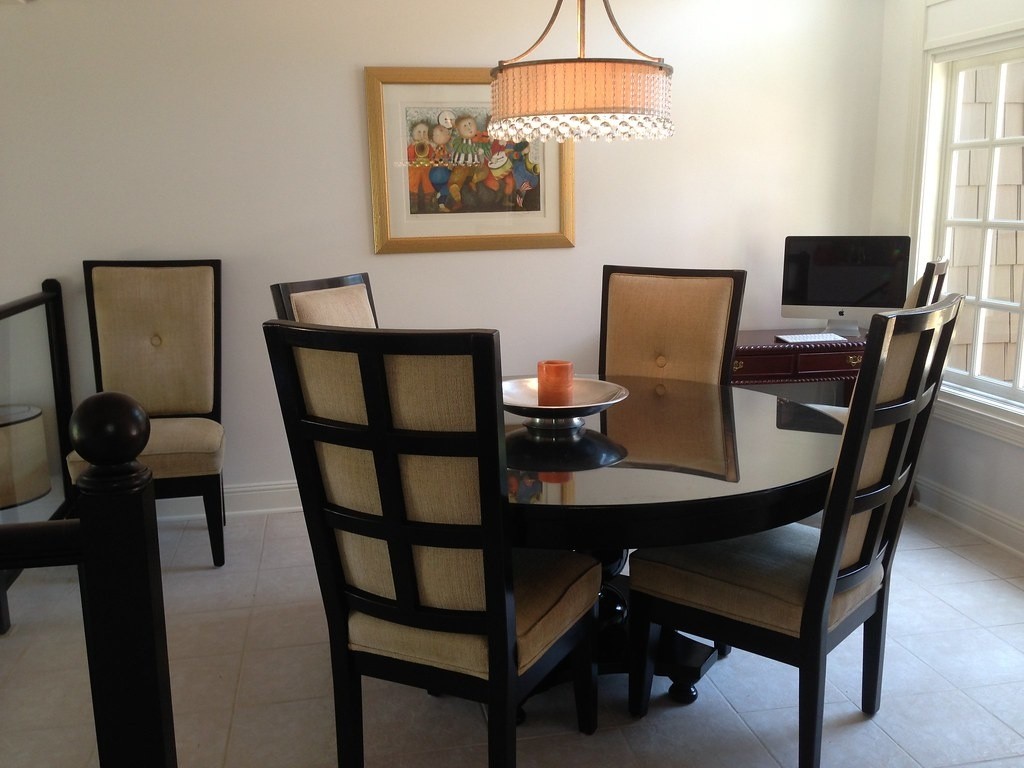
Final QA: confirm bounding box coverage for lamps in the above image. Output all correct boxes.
[487,0,675,147]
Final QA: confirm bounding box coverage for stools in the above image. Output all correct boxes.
[0,403,52,510]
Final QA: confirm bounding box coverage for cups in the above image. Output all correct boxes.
[538,360,574,406]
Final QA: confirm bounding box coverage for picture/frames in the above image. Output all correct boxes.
[364,66,577,255]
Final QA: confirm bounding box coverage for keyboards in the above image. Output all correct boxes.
[774,332,848,344]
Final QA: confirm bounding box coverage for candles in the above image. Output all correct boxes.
[538,360,572,407]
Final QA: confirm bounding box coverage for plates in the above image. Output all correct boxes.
[502,378,629,429]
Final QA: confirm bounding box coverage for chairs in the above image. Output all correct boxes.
[260,255,968,768]
[66,259,227,568]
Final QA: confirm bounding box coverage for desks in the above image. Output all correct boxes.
[728,328,918,509]
[500,375,846,702]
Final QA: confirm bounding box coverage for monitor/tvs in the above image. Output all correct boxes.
[781,235,911,337]
